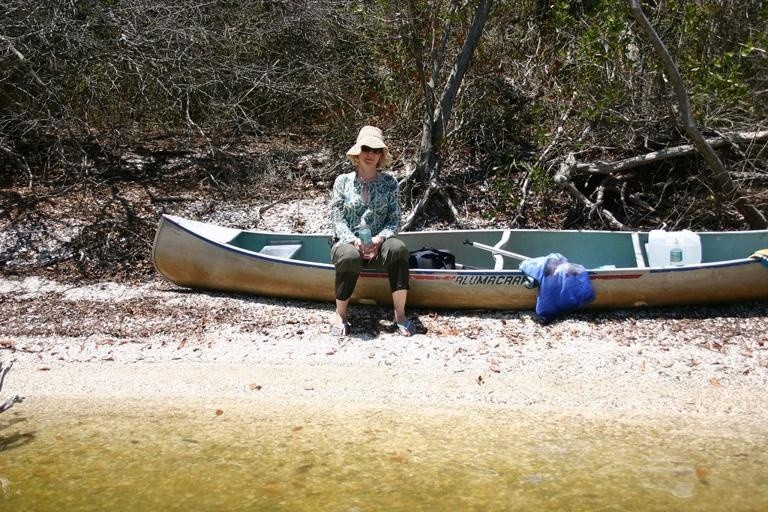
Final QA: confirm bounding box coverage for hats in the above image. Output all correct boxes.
[346,126,392,168]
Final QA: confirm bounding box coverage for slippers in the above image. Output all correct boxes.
[397,321,416,336]
[335,324,352,336]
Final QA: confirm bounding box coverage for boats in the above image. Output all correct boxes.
[151,213,768,311]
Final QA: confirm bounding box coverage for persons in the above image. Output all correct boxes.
[324,125,416,337]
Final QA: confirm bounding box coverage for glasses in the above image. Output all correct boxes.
[362,146,383,153]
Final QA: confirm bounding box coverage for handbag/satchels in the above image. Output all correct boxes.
[409,245,455,269]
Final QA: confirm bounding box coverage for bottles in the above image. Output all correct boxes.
[360,219,372,247]
[671,240,682,263]
